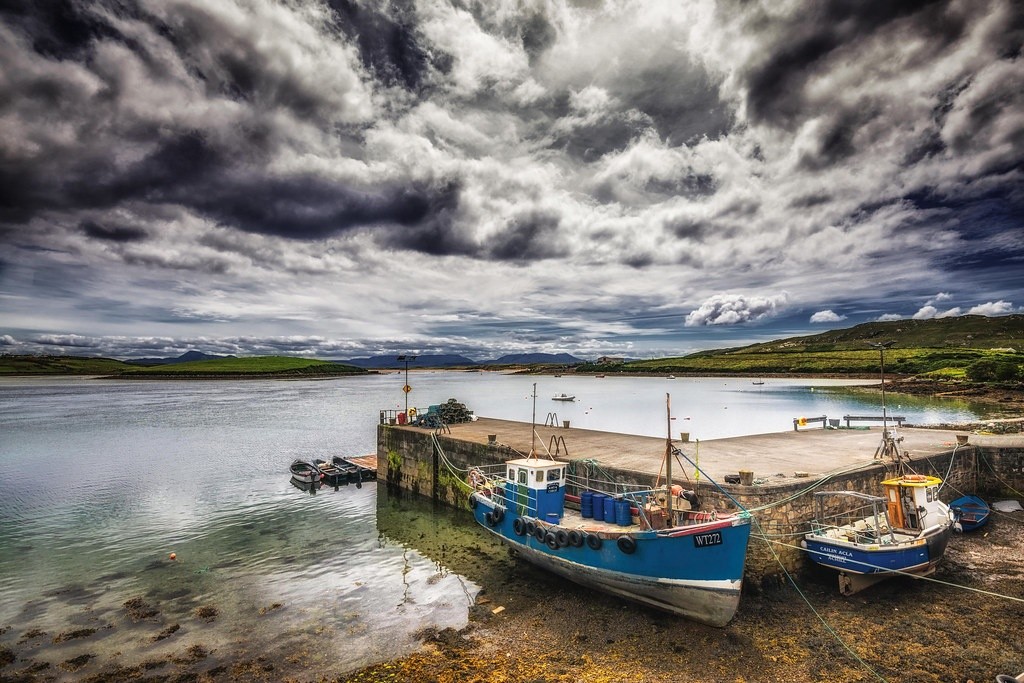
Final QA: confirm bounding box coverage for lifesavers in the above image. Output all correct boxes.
[586,533,601,549]
[470,471,480,486]
[493,507,503,523]
[545,532,559,550]
[513,517,526,536]
[469,496,476,509]
[555,529,569,547]
[402,385,411,392]
[527,522,536,536]
[568,530,583,547]
[408,408,416,416]
[616,535,636,554]
[535,526,546,543]
[486,512,496,527]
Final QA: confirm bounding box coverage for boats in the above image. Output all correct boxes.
[804,472,957,596]
[312,457,347,482]
[949,494,990,532]
[596,374,605,378]
[555,374,561,377]
[323,479,347,491]
[289,476,321,492]
[289,459,320,482]
[468,384,752,629]
[550,393,575,401]
[332,455,369,481]
[666,375,675,380]
[752,381,764,385]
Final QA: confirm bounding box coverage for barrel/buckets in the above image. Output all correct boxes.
[581,492,593,518]
[545,513,559,525]
[592,493,607,521]
[398,412,406,425]
[614,498,631,526]
[603,496,616,524]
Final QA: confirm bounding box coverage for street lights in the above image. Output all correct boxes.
[397,354,417,423]
[866,340,898,454]
